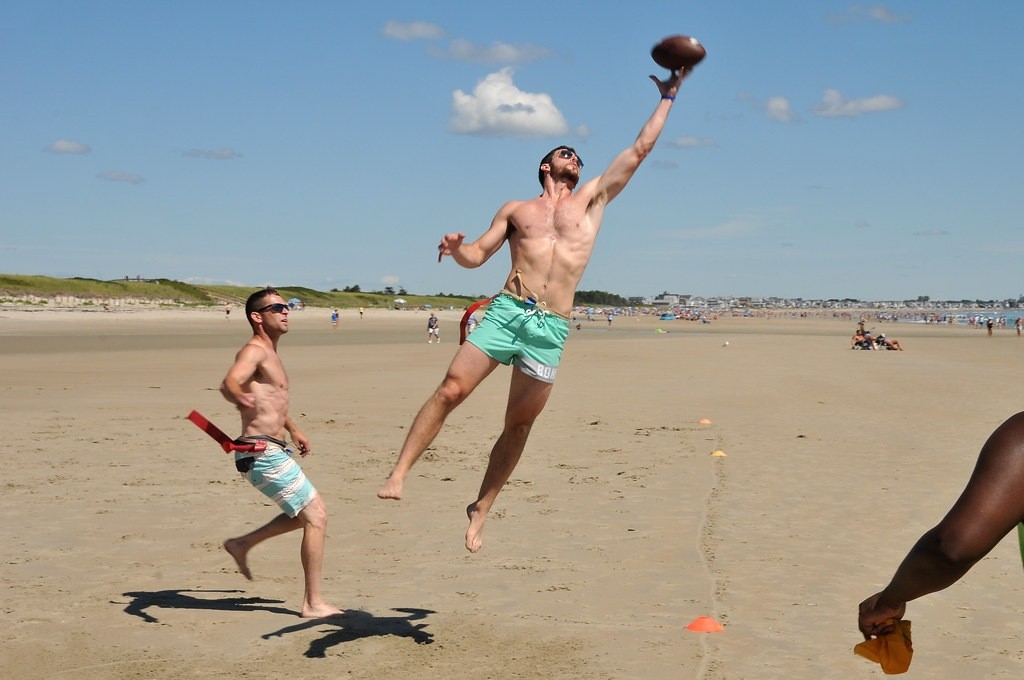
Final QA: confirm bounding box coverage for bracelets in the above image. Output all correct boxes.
[661,94,676,102]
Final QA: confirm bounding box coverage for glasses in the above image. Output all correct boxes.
[552,150,584,170]
[257,303,290,313]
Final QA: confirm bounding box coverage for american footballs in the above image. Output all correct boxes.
[650,35,706,71]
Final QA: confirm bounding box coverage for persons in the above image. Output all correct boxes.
[217,287,348,620]
[571,302,1024,352]
[225,302,233,319]
[393,295,468,313]
[359,306,364,319]
[857,410,1024,642]
[334,309,339,326]
[332,311,337,327]
[468,313,478,336]
[285,297,305,311]
[377,64,691,555]
[426,312,441,345]
